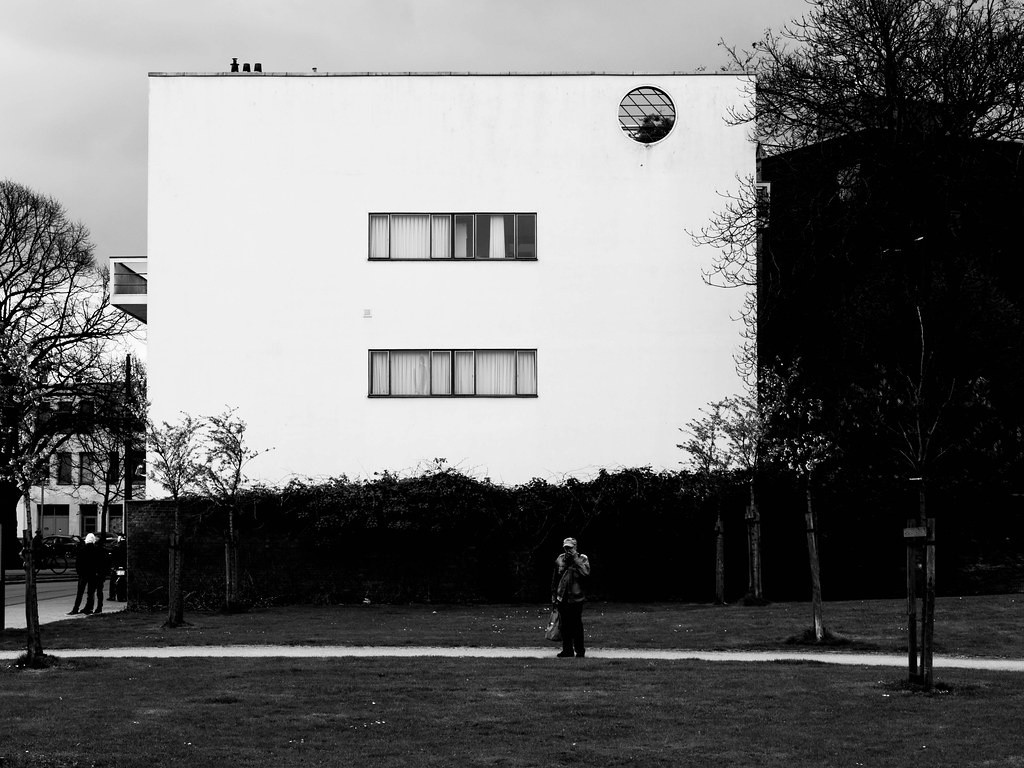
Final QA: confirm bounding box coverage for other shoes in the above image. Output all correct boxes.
[81,609,93,613]
[94,607,102,613]
[68,610,78,615]
[576,653,584,657]
[557,651,574,657]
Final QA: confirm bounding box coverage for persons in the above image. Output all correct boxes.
[31,530,44,568]
[552,537,590,657]
[67,534,126,615]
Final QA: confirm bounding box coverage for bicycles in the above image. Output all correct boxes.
[19,541,69,575]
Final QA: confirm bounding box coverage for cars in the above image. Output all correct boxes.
[42,535,83,565]
[77,530,119,550]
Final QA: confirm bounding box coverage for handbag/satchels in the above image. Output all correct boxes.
[545,606,562,641]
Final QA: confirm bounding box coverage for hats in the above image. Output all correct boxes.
[562,537,577,548]
